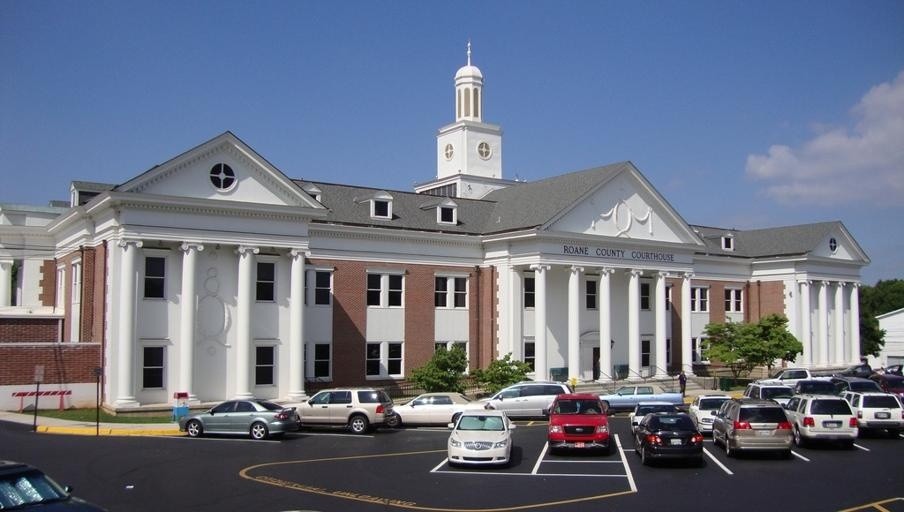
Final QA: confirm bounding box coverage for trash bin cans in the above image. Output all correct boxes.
[720,378,730,391]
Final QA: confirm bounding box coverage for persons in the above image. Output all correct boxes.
[678,371,687,397]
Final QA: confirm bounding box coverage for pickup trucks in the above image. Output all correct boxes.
[599,382,684,413]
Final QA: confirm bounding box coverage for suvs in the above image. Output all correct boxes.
[541,392,614,454]
[293,385,398,435]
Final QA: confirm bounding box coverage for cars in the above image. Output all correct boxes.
[176,398,302,441]
[0,458,108,512]
[626,400,685,435]
[383,391,493,428]
[689,360,904,457]
[630,412,704,468]
[443,408,517,467]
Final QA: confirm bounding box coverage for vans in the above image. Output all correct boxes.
[478,379,574,421]
